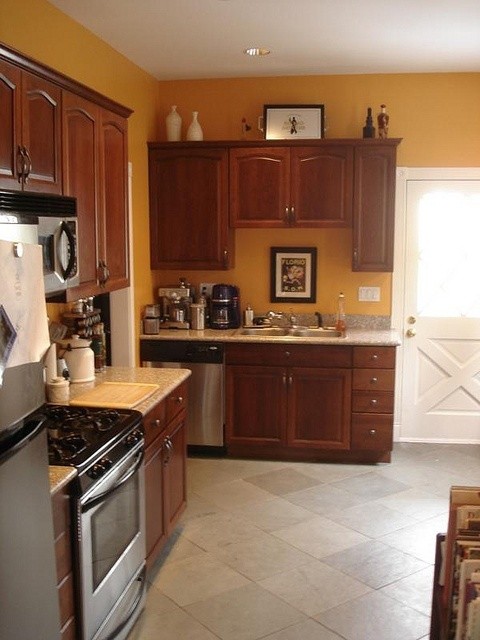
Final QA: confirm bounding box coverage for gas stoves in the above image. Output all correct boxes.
[46,403,146,494]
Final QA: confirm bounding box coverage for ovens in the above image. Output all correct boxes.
[68,437,148,640]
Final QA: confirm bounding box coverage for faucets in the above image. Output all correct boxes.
[288,307,297,328]
[314,312,322,327]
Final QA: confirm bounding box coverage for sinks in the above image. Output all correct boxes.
[294,330,346,337]
[240,328,288,336]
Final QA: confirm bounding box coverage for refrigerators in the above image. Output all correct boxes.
[0,239,50,640]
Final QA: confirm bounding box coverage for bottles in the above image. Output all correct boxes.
[378,104,388,138]
[165,105,181,141]
[186,112,203,141]
[336,294,346,333]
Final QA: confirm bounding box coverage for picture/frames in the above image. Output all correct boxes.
[270,246,317,303]
[258,102,330,140]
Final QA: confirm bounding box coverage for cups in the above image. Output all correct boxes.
[190,303,206,330]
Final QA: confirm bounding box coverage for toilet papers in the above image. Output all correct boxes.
[44,343,57,378]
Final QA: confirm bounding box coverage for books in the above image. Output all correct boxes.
[438,484,480,640]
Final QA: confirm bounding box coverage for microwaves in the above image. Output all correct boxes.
[0,187,80,293]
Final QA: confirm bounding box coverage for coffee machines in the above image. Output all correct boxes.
[211,284,240,330]
[158,277,192,330]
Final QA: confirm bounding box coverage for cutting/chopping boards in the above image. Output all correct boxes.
[68,381,160,410]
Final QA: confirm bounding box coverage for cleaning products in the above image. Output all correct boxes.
[336,292,347,329]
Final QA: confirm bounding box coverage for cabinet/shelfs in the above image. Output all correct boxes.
[229,139,354,229]
[146,139,236,271]
[143,375,190,571]
[352,341,400,464]
[1,45,63,193]
[352,137,404,273]
[52,488,79,640]
[225,338,353,464]
[64,72,134,303]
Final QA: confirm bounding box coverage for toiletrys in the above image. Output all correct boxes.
[245,304,253,325]
